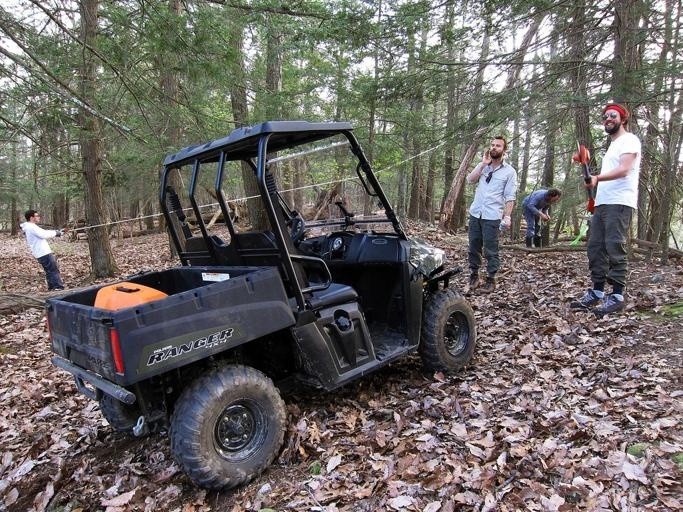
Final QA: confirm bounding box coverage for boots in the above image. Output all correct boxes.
[468,273,479,289]
[525,236,532,247]
[534,235,540,247]
[481,276,495,294]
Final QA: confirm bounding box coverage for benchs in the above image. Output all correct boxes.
[183,230,358,310]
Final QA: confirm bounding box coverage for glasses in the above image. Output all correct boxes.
[603,111,618,120]
[486,172,492,183]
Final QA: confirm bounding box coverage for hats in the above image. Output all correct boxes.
[603,103,629,120]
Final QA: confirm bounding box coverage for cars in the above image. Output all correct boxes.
[45,120,477,489]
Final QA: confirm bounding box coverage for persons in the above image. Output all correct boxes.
[519,187,563,249]
[22,209,64,290]
[568,103,642,317]
[465,137,519,296]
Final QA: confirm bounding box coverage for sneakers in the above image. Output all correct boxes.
[591,294,625,316]
[576,287,605,308]
